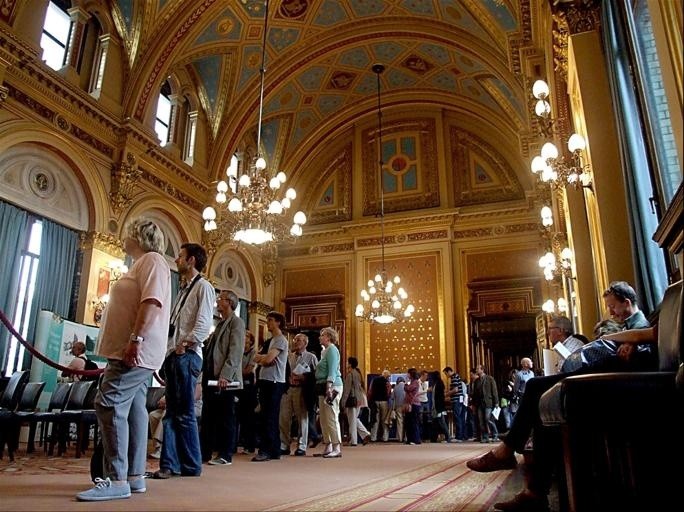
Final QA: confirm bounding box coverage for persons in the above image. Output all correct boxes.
[341,356,535,443]
[77,219,172,502]
[465,282,658,512]
[147,243,344,477]
[61,342,88,382]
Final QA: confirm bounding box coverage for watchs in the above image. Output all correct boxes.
[130,333,144,342]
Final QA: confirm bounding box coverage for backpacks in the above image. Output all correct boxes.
[78,356,100,389]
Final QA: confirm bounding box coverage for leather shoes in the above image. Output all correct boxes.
[324,452,342,458]
[494,490,550,511]
[467,450,518,472]
[313,452,331,456]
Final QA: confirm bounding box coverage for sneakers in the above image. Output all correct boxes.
[128,477,147,494]
[148,448,162,459]
[252,453,280,461]
[209,456,233,466]
[343,434,503,447]
[76,476,131,500]
[281,449,291,456]
[295,449,306,456]
[154,468,176,478]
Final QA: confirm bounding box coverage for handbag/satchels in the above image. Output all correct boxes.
[345,396,357,409]
[561,338,617,373]
[391,410,396,420]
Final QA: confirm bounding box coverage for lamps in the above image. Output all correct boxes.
[108,260,128,284]
[355,64,414,323]
[531,79,593,312]
[90,295,108,309]
[200,1,306,250]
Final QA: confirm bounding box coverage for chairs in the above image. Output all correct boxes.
[537,280,682,509]
[0,369,164,463]
